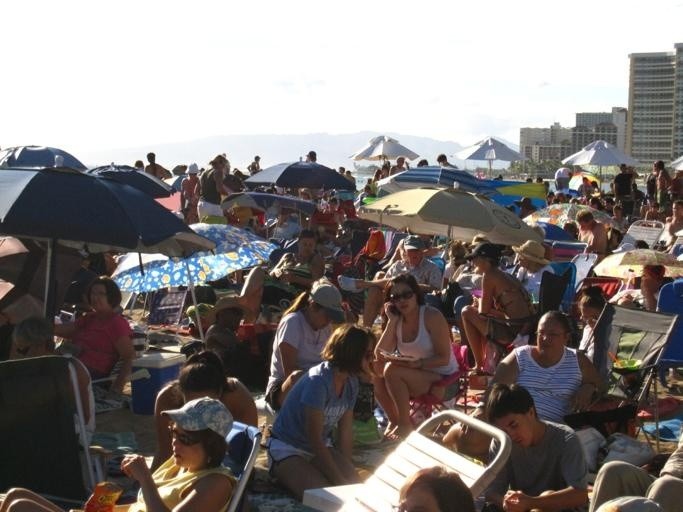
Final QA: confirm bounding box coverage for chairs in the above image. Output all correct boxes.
[0,219,683,512]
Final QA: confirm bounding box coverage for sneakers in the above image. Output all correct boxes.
[338,275,364,293]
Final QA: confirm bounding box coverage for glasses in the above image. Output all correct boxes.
[388,291,413,302]
[168,425,200,446]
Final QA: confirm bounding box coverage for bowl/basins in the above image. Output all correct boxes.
[612,358,643,371]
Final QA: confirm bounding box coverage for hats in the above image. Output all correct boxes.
[160,397,234,439]
[515,197,535,209]
[512,240,551,265]
[207,297,256,323]
[184,163,200,174]
[404,235,425,251]
[300,188,311,199]
[309,281,346,323]
[468,234,490,248]
[464,243,498,260]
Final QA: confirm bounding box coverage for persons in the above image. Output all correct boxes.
[0,150,683,512]
